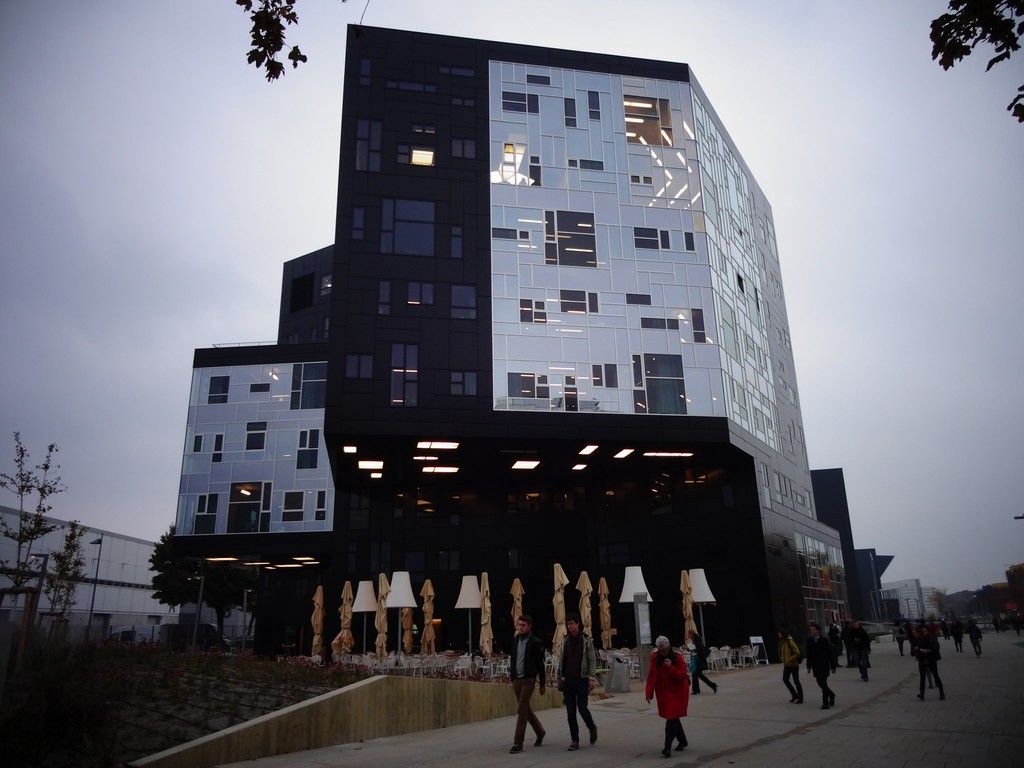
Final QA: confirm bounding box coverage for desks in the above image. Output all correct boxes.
[482,657,503,679]
[724,648,747,669]
[622,652,639,679]
[444,657,461,679]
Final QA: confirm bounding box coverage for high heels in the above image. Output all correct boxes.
[691,690,700,695]
[713,683,718,694]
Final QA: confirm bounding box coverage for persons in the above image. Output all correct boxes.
[806,624,836,710]
[778,629,804,705]
[645,636,689,756]
[910,628,945,700]
[688,630,718,695]
[828,619,871,682]
[508,616,546,754]
[558,614,597,751]
[892,607,1021,690]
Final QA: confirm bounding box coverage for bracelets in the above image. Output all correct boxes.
[589,679,595,681]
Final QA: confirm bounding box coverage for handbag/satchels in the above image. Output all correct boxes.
[899,629,905,635]
[795,650,804,664]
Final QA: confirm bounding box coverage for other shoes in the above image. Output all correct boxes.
[929,682,933,689]
[661,748,672,755]
[509,741,523,753]
[534,731,546,746]
[821,704,830,710]
[939,695,945,700]
[976,654,983,658]
[863,676,868,682]
[675,741,688,751]
[567,739,579,750]
[794,698,804,704]
[590,727,597,744]
[830,694,835,706]
[917,694,924,701]
[789,695,800,702]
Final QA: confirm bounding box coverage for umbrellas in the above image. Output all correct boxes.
[311,562,701,656]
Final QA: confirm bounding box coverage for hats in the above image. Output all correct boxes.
[655,636,670,650]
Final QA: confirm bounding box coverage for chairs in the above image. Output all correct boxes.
[303,645,760,679]
